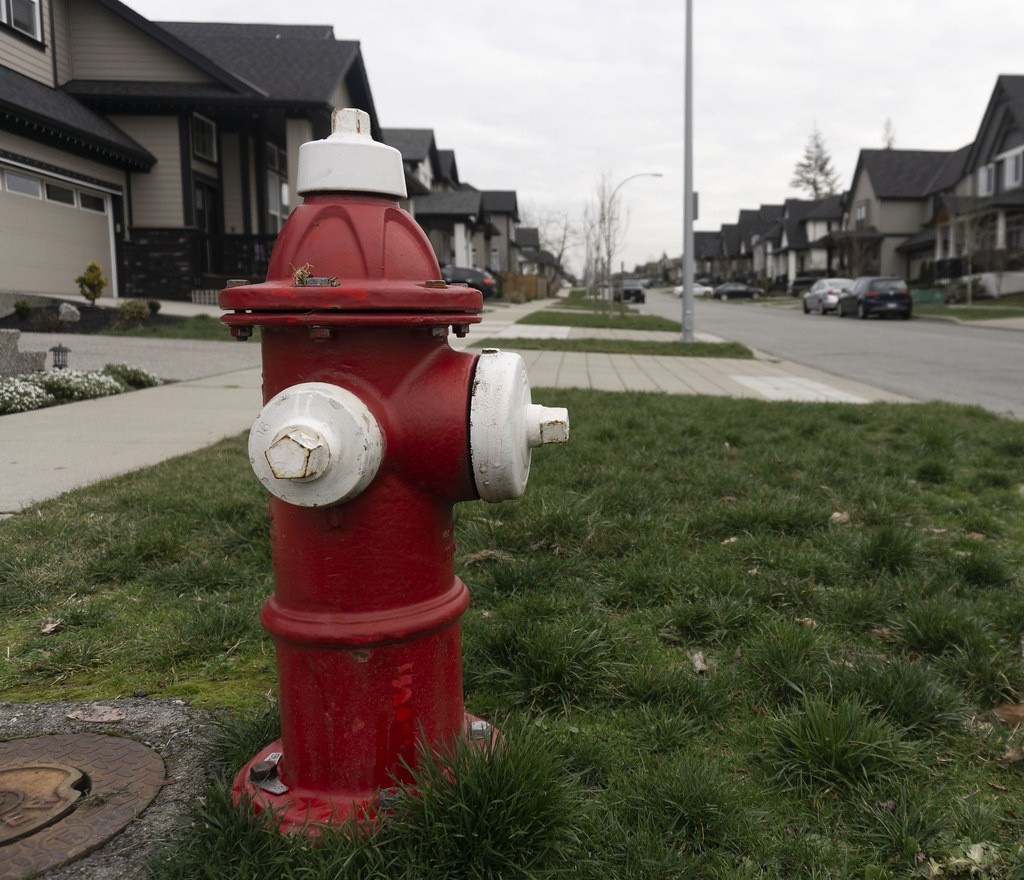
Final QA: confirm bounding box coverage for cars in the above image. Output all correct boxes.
[637,277,665,290]
[836,276,913,321]
[673,283,714,296]
[713,282,768,301]
[803,277,853,315]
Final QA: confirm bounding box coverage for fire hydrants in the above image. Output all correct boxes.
[218,104,573,848]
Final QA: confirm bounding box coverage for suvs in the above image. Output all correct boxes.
[440,264,497,301]
[612,279,646,303]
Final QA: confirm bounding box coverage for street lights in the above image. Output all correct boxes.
[606,173,662,319]
